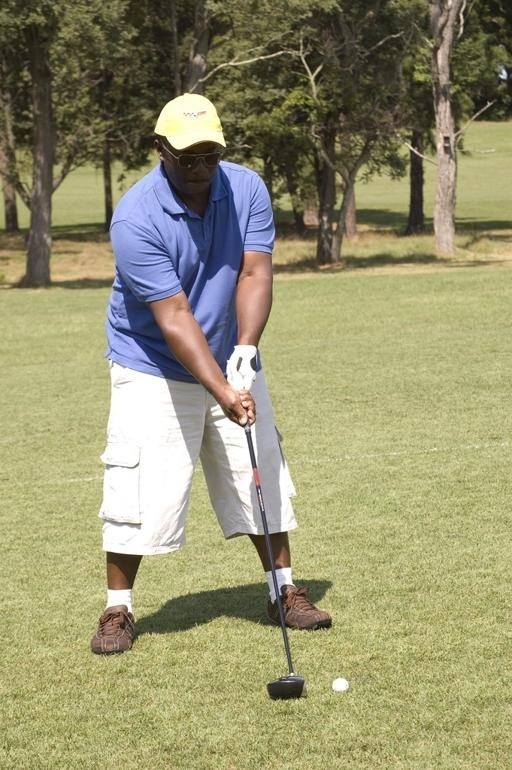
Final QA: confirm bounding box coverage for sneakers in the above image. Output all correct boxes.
[268,585,332,630]
[90,604,137,654]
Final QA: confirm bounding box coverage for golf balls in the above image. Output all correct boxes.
[332,678,347,690]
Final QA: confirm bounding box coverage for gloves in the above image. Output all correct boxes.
[225,344,261,393]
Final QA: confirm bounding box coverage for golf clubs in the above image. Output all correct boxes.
[243,421,305,700]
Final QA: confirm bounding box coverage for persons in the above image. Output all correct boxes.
[90,93,335,656]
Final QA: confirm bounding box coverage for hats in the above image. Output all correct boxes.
[154,93,227,151]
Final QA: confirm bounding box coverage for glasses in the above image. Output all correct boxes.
[162,142,222,169]
